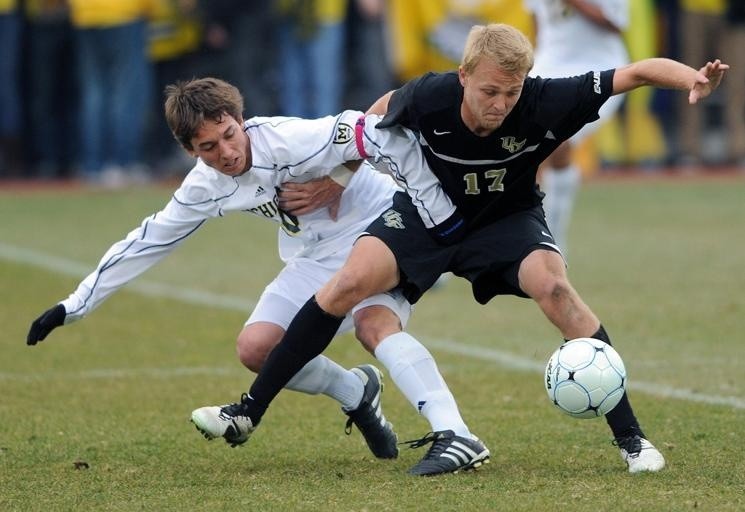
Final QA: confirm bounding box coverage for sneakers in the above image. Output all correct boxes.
[406,429,490,477]
[191,392,261,448]
[341,363,398,457]
[611,435,666,472]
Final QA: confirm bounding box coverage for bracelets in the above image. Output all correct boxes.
[327,164,356,190]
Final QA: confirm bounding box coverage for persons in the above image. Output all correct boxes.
[28,73,492,478]
[519,0,635,259]
[190,21,731,472]
[601,1,744,170]
[1,1,534,185]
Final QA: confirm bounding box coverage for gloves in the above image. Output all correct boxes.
[27,304,66,346]
[430,209,469,247]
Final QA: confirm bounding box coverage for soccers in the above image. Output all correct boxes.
[544,338,626,418]
